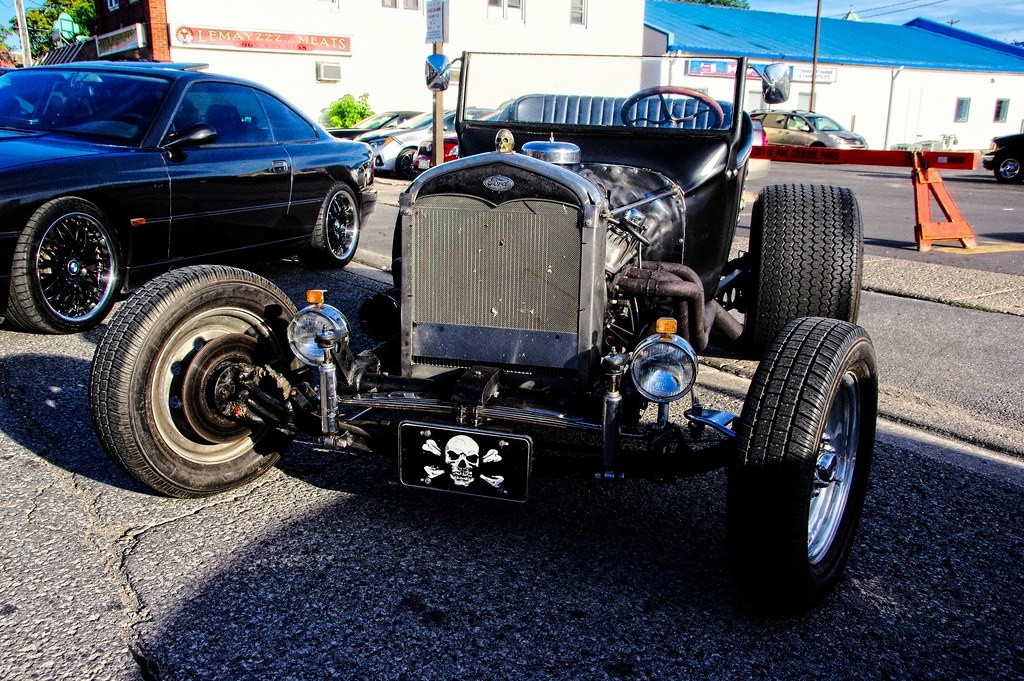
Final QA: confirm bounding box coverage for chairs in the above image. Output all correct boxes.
[204,104,264,144]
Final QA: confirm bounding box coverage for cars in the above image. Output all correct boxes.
[88,38,881,604]
[0,58,380,334]
[983,129,1024,182]
[333,107,500,181]
[748,105,869,150]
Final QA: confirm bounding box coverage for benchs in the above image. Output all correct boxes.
[510,95,735,130]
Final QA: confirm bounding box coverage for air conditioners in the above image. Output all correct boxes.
[317,61,342,82]
